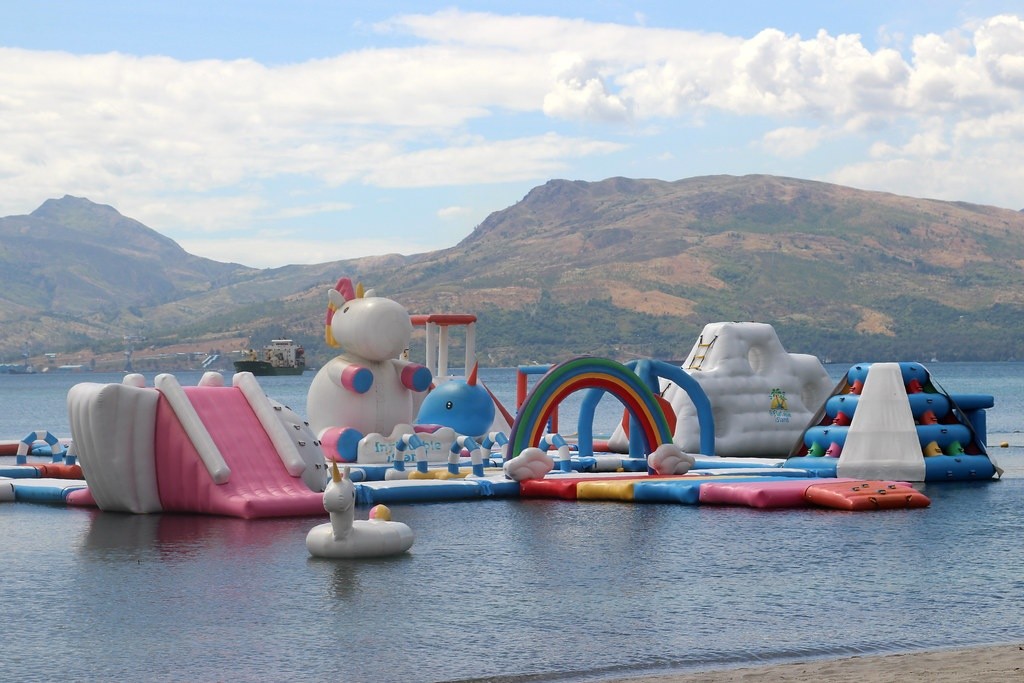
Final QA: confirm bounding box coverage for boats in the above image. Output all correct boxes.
[234,340,306,376]
